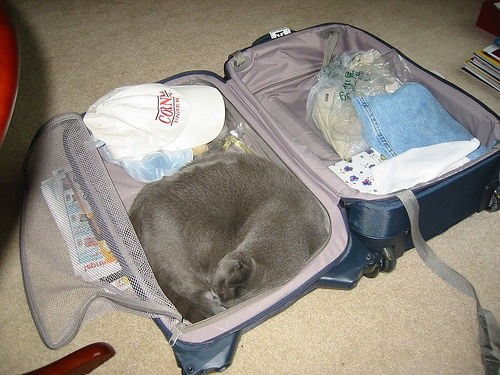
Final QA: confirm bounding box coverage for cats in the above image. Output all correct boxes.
[127,153,330,323]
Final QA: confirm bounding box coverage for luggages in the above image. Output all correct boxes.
[19,22,499,375]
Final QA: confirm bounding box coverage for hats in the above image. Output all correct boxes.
[83,82,226,161]
